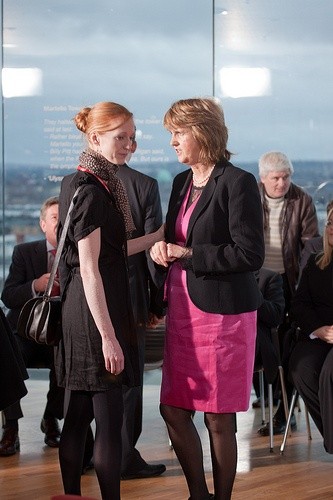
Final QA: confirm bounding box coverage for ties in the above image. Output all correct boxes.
[48,249,60,296]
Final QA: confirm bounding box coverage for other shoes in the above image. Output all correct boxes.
[188,494,214,500]
[252,397,278,408]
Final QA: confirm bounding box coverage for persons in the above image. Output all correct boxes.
[150,99,266,500]
[290,205,333,453]
[258,200,333,435]
[0,306,29,412]
[83,127,167,481]
[0,197,64,456]
[57,101,165,498]
[252,154,317,406]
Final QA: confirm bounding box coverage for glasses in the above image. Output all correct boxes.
[325,221,333,227]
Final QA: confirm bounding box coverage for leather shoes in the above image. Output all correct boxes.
[257,415,297,436]
[41,417,61,447]
[119,448,166,479]
[0,436,20,456]
[84,462,94,470]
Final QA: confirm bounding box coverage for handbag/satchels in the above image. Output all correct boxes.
[16,296,62,345]
